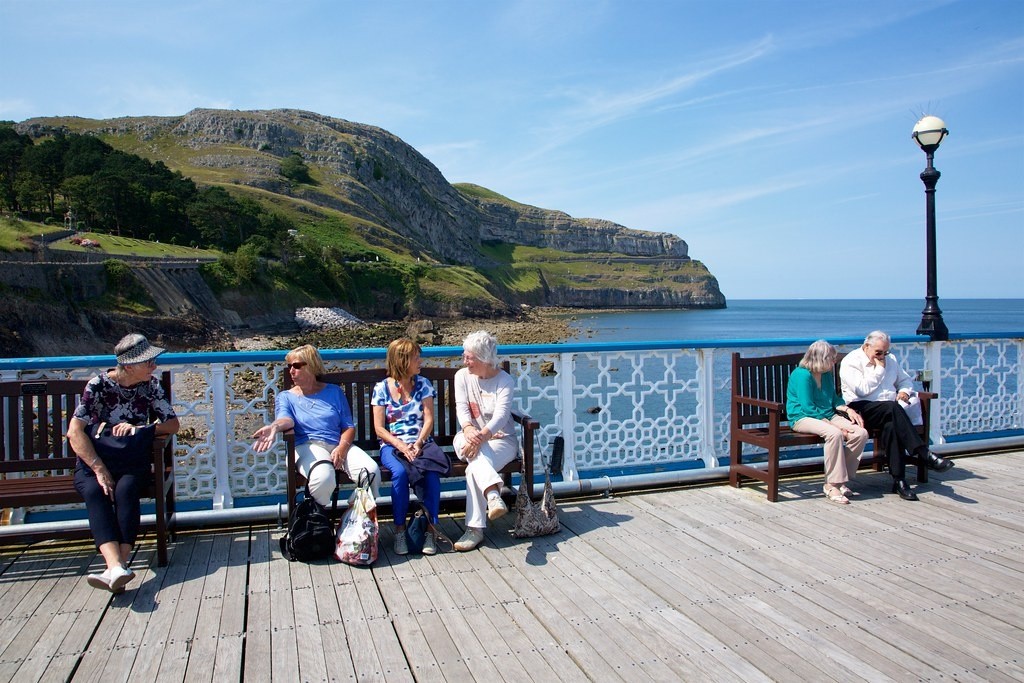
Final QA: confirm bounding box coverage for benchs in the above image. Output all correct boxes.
[729,352,938,502]
[0,370,177,569]
[283,362,540,532]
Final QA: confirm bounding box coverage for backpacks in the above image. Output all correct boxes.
[279,460,335,565]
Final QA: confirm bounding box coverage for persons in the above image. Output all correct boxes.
[253,344,381,510]
[67,333,179,593]
[786,339,869,503]
[452,330,518,550]
[371,338,441,554]
[839,330,954,500]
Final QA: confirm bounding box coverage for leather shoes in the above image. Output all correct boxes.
[893,480,917,500]
[927,452,955,473]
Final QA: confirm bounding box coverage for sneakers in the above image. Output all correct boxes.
[454,528,483,551]
[88,566,136,593]
[487,496,508,520]
[394,531,408,554]
[422,533,437,554]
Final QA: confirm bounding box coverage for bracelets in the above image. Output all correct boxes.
[846,407,849,412]
[463,425,473,432]
[90,456,98,468]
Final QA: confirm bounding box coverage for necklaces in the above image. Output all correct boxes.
[298,382,321,410]
[395,379,415,405]
[115,372,137,400]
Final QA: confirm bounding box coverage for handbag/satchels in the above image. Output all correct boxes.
[514,476,561,538]
[407,502,453,555]
[335,467,379,565]
[87,422,156,457]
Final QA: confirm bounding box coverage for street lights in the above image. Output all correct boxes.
[911,115,949,335]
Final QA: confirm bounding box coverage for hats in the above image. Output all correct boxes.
[117,339,167,364]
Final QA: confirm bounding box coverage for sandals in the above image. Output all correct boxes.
[823,484,852,504]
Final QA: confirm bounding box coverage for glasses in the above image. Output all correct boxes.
[871,347,891,356]
[144,359,157,367]
[288,362,307,369]
[462,354,473,361]
